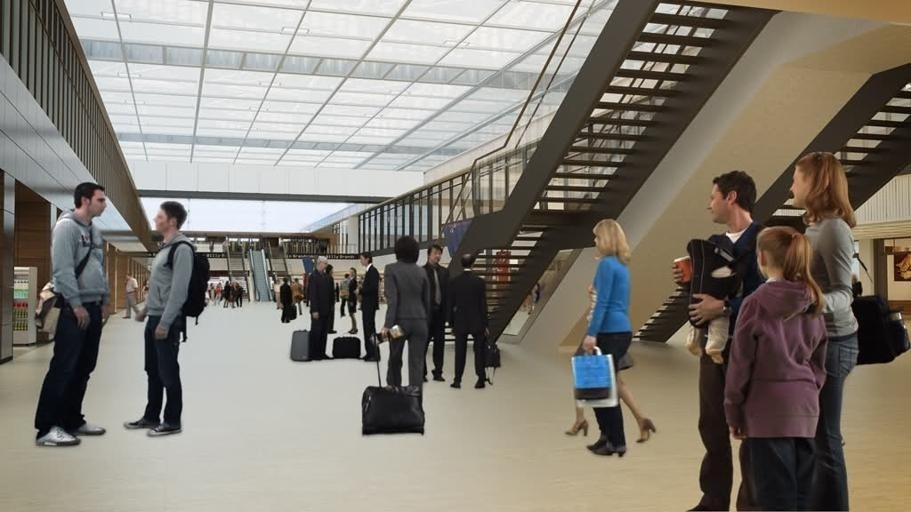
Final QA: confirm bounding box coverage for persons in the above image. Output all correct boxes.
[34,182,110,446]
[123,202,197,436]
[208,280,244,308]
[724,226,829,511]
[141,279,150,303]
[121,273,141,319]
[380,235,488,408]
[307,252,381,361]
[565,370,655,443]
[672,171,769,511]
[790,151,859,511]
[272,276,304,323]
[574,219,632,457]
[207,236,328,254]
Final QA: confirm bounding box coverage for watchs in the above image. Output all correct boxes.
[723,300,731,314]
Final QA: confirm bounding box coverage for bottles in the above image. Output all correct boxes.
[13,278,28,331]
[889,309,909,349]
